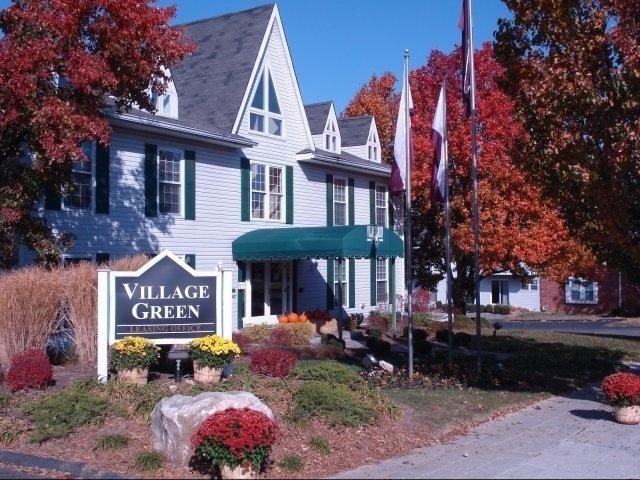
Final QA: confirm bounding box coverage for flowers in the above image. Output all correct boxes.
[191,334,241,367]
[108,335,160,369]
[599,371,640,406]
[185,407,279,470]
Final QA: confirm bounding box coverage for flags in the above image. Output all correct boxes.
[428,84,448,204]
[457,3,478,118]
[387,83,414,195]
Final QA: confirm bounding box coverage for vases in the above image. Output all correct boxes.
[114,362,150,385]
[193,361,224,384]
[614,404,640,425]
[217,457,263,479]
